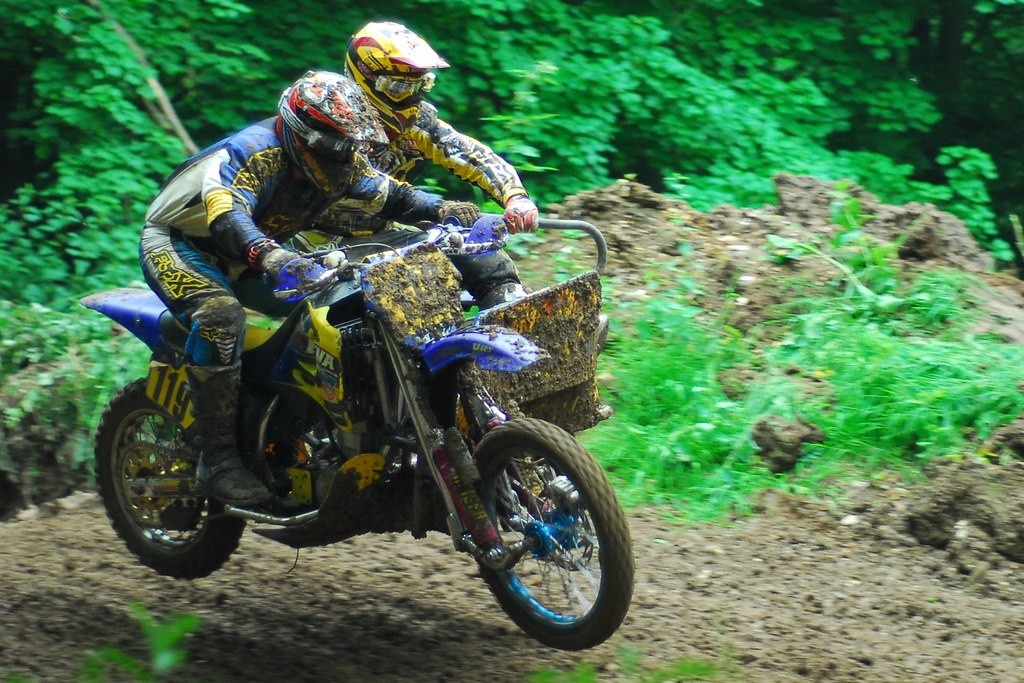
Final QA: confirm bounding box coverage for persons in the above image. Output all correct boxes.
[137,73,484,508]
[284,21,609,311]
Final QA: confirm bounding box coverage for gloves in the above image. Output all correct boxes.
[263,250,301,282]
[503,194,543,236]
[437,199,485,228]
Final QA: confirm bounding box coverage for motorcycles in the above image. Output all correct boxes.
[80,205,638,652]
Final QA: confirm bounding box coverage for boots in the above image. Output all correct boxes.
[183,360,269,508]
[484,283,610,358]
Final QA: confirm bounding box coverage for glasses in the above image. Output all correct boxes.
[373,74,428,102]
[340,136,372,165]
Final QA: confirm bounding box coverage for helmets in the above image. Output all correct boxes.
[273,71,391,199]
[344,21,451,138]
